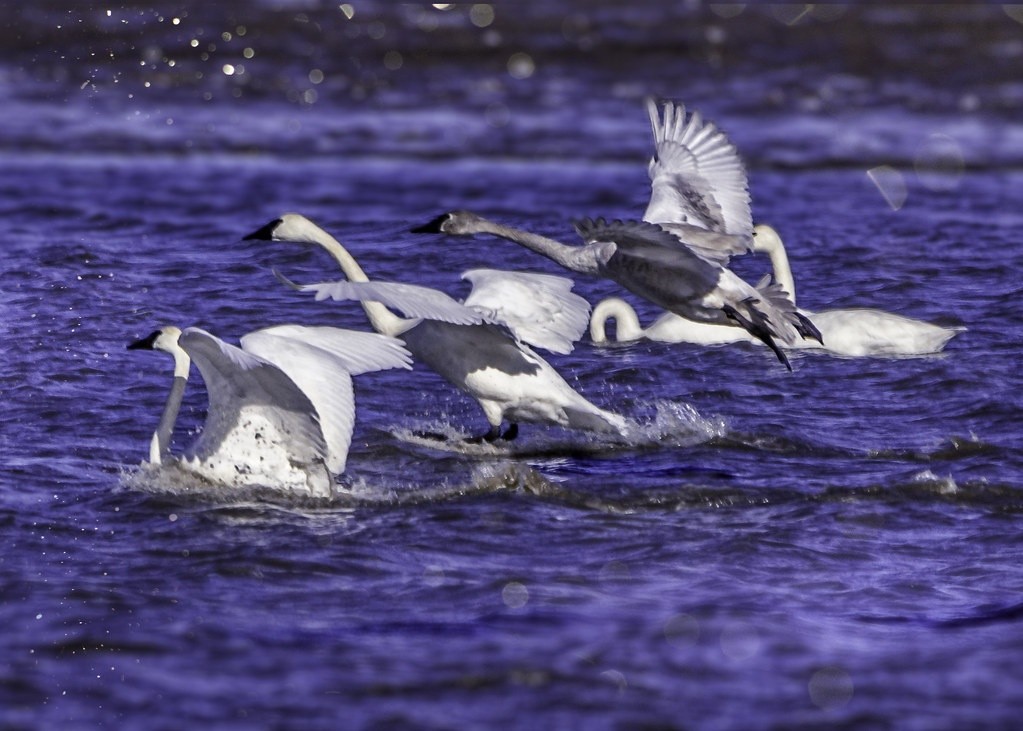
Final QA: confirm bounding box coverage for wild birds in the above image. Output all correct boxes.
[130,94,970,499]
